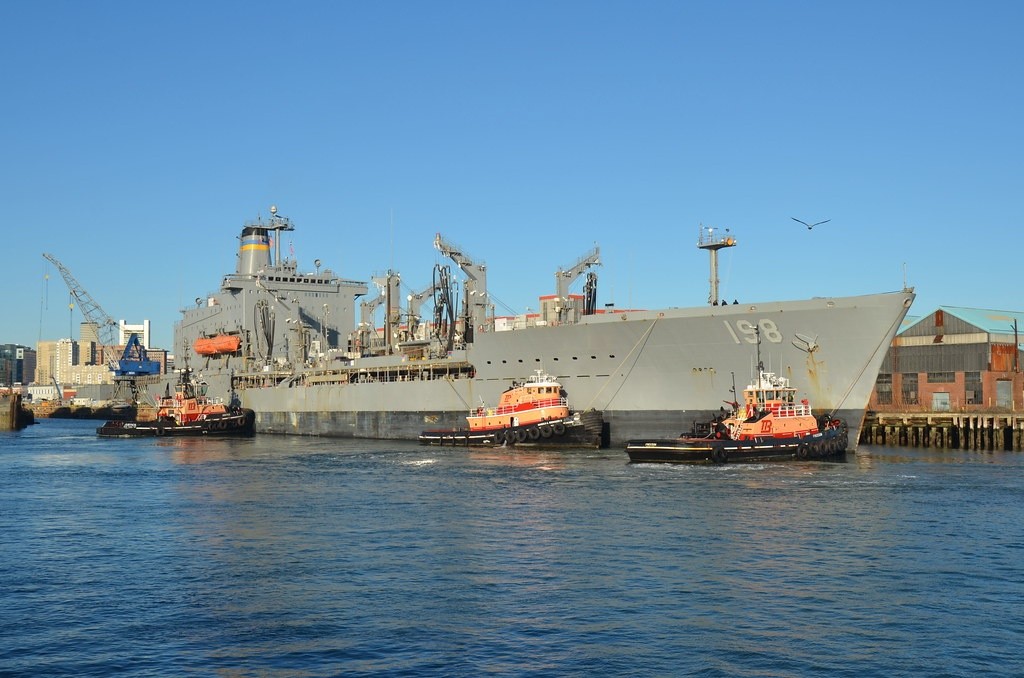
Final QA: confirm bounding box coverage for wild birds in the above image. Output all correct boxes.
[792,216,830,229]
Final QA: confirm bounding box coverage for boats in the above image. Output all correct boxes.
[624,327,857,461]
[73,207,917,456]
[418,369,605,452]
[96,340,255,438]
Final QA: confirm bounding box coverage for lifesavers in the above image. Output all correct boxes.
[797,444,810,460]
[238,417,246,425]
[156,427,165,436]
[528,426,540,439]
[553,423,566,434]
[541,425,553,438]
[209,420,238,430]
[493,430,505,444]
[515,429,527,441]
[712,446,729,463]
[809,442,820,457]
[819,432,849,457]
[505,430,516,443]
